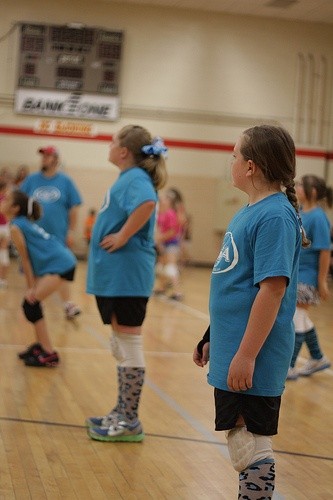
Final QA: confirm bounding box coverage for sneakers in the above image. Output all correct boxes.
[64,304,82,320]
[17,343,61,367]
[86,411,143,442]
[287,368,298,380]
[298,355,331,376]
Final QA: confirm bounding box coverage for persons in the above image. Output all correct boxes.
[153,188,190,302]
[87,124,173,443]
[193,124,312,500]
[286,174,333,380]
[17,145,82,320]
[0,187,79,368]
[85,207,96,245]
[0,165,30,289]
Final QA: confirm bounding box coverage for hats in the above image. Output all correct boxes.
[37,145,57,156]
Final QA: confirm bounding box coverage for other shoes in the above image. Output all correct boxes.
[0,280,9,289]
[151,290,166,297]
[167,293,184,302]
